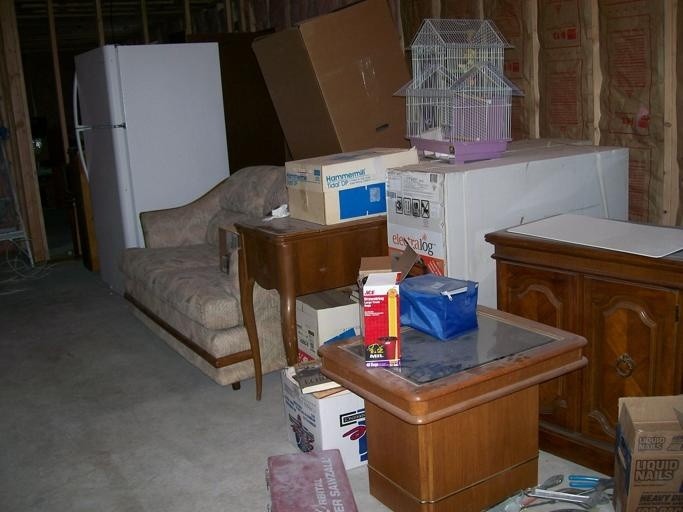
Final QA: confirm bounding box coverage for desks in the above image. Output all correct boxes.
[229,216,385,401]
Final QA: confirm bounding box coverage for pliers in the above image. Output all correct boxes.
[568,474,617,488]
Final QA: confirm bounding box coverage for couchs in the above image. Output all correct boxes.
[117,161,288,392]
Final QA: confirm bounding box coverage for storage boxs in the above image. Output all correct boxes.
[250,3,629,473]
[610,395,683,512]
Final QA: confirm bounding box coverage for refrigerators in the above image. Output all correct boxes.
[71,42,232,296]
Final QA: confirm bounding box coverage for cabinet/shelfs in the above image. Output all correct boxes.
[483,212,683,479]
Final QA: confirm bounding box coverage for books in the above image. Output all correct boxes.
[350,290,360,303]
[292,357,347,399]
